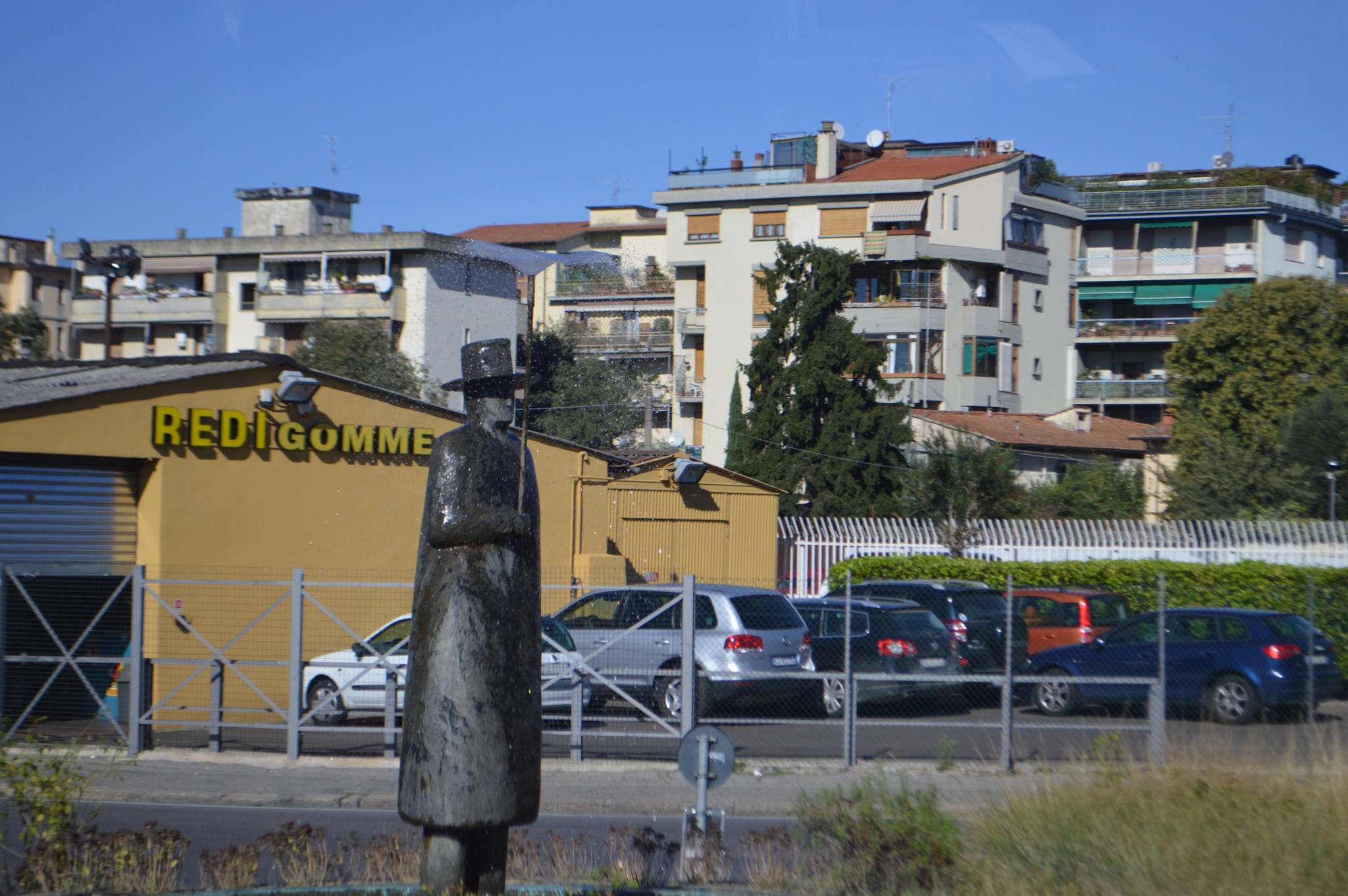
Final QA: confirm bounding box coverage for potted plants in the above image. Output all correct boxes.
[1077,323,1184,338]
[1224,262,1252,273]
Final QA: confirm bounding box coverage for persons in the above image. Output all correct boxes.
[398,338,543,896]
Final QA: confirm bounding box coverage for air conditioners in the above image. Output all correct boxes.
[997,140,1015,153]
[1213,156,1228,169]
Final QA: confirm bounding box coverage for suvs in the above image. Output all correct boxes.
[785,595,969,718]
[1016,605,1344,727]
[549,582,823,723]
[824,577,1029,710]
[996,587,1136,658]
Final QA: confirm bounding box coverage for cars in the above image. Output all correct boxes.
[302,609,593,727]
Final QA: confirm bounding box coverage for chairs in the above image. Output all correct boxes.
[1174,622,1194,641]
[1023,603,1044,627]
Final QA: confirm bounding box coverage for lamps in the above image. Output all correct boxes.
[672,458,708,484]
[276,370,322,404]
